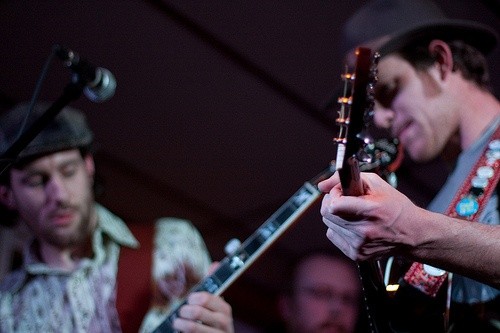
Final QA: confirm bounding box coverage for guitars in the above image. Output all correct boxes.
[146,132,407,333]
[335,45,443,333]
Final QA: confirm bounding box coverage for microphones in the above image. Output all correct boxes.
[56,47,116,102]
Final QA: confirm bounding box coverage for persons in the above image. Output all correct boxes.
[279,247,361,333]
[321,0,500,333]
[1,104,235,332]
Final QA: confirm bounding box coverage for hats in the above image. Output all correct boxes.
[0,102,93,160]
[341,0,495,69]
